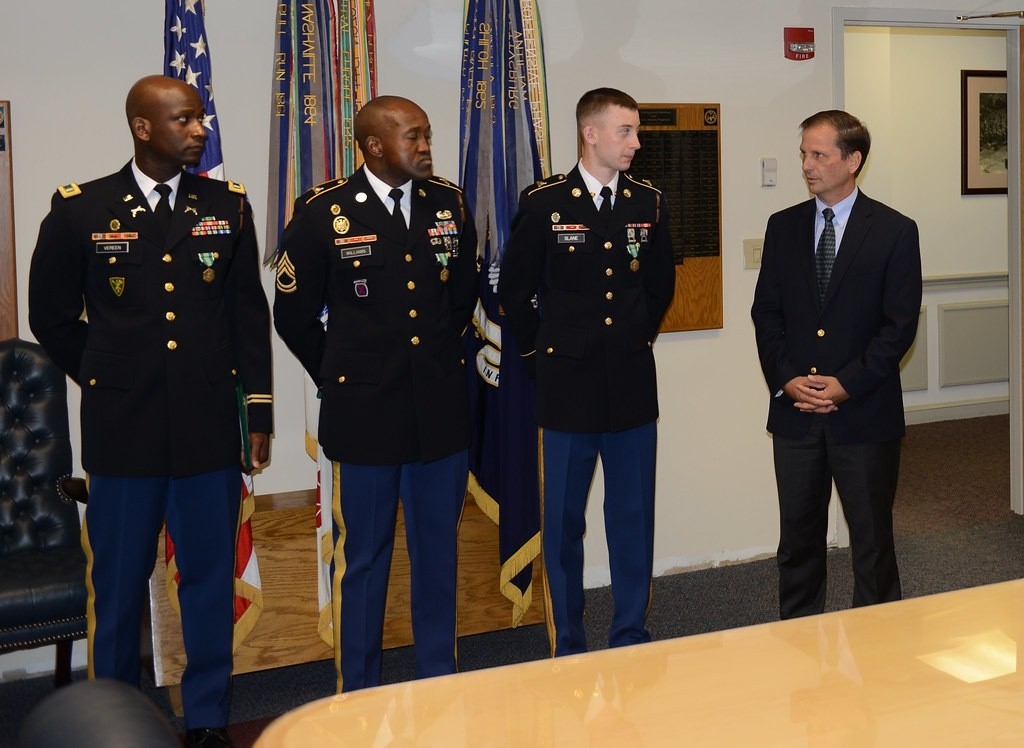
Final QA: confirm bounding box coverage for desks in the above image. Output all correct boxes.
[252,575,1024,748]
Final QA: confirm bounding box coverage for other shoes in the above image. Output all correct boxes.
[186,727,233,748]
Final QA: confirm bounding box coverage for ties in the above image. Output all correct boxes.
[816,208,836,310]
[388,189,407,242]
[153,184,173,242]
[599,187,612,230]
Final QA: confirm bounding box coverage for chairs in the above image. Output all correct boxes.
[1,336,91,682]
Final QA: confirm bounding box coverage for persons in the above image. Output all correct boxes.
[274,95,479,693]
[750,110,923,620]
[497,88,677,657]
[29,76,273,748]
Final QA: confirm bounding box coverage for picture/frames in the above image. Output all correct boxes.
[961,69,1008,194]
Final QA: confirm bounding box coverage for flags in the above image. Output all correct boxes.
[165,0,264,652]
[460,1,552,628]
[265,0,377,648]
